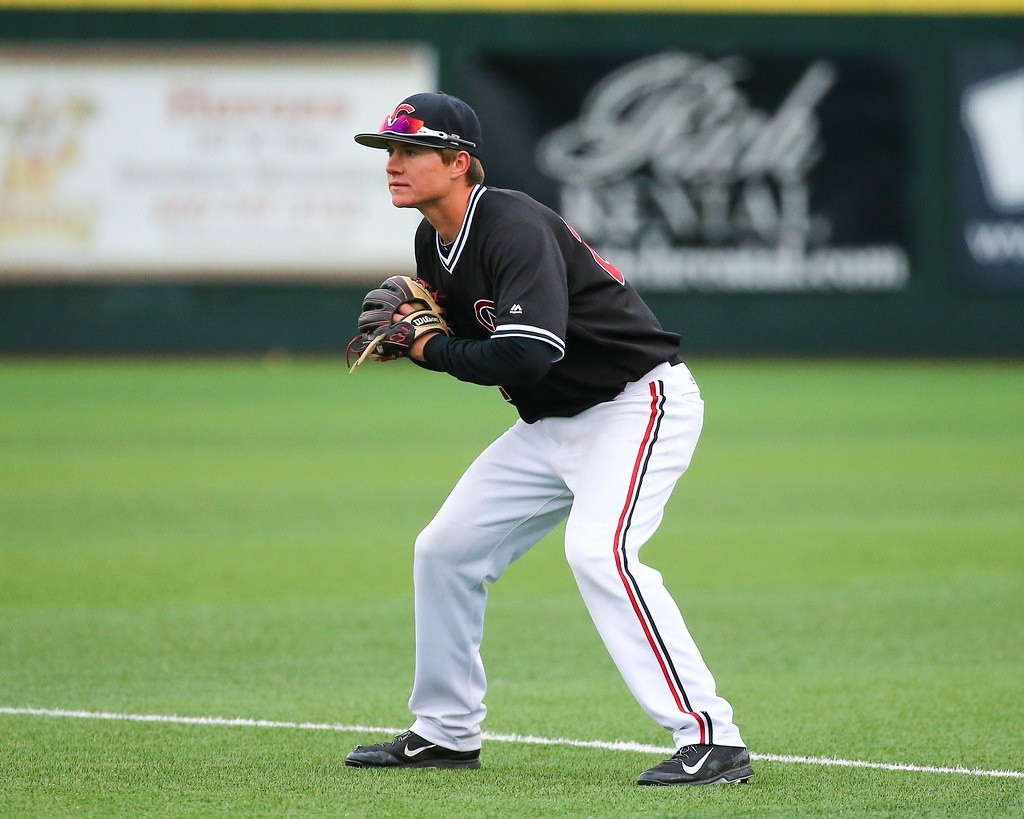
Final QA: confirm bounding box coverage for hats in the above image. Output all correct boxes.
[354,91,485,160]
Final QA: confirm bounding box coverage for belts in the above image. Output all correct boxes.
[669,353,685,367]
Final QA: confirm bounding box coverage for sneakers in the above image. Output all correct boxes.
[636,742,755,786]
[344,730,483,769]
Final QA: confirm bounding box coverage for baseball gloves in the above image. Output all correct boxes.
[356,274,450,363]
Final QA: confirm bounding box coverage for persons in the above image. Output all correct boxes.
[344,92,752,787]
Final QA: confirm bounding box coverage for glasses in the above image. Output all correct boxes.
[377,116,478,148]
